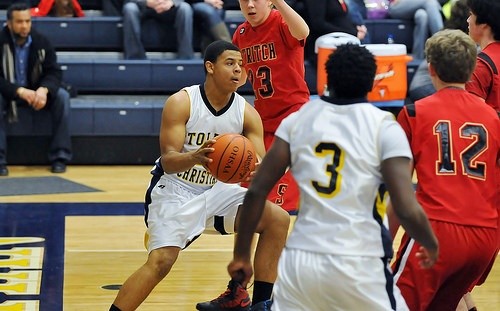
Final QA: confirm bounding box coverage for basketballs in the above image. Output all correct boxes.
[207,135,256,183]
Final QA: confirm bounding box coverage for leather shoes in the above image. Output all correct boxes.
[51,160,66,172]
[0,164,9,176]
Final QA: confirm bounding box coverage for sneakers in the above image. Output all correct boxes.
[252,299,273,311]
[196,279,251,311]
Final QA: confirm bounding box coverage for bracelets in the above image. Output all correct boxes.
[20,88,25,98]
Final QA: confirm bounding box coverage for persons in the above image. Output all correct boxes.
[284,0,368,73]
[109,40,291,311]
[456,0,500,311]
[386,29,500,311]
[0,3,73,176]
[227,41,440,311]
[104,0,195,61]
[388,0,445,60]
[195,0,310,311]
[184,0,233,59]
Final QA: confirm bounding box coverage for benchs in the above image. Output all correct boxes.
[5,0,420,165]
